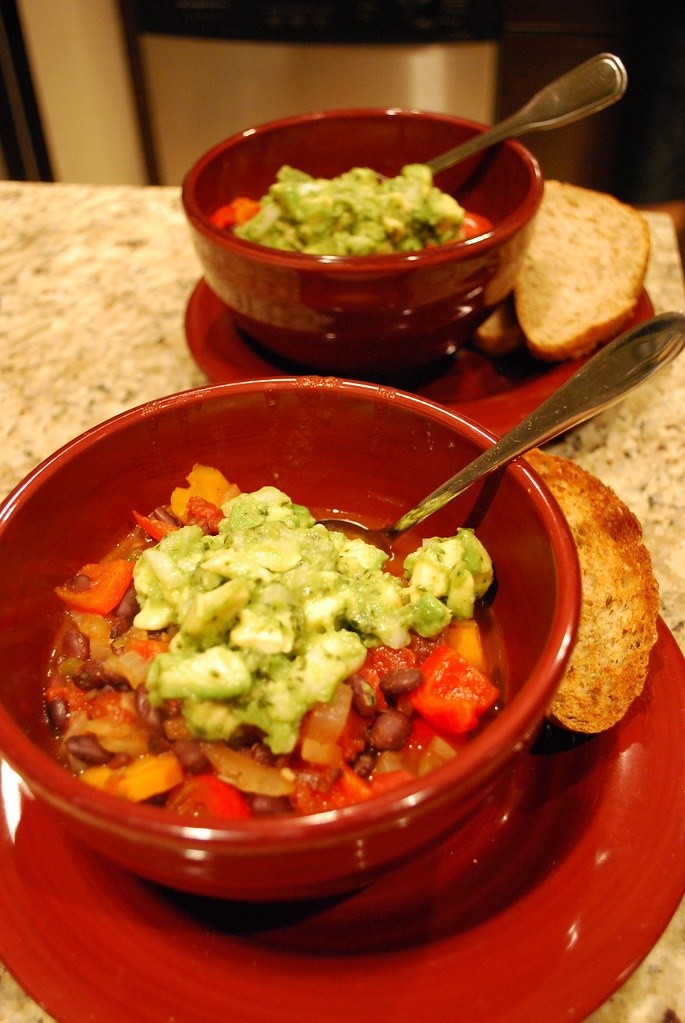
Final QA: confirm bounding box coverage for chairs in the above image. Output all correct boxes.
[131,30,498,184]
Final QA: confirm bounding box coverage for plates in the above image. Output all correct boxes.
[0,616,685,1021]
[184,272,655,441]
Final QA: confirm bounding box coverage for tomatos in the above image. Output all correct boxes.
[49,513,499,820]
[213,197,492,236]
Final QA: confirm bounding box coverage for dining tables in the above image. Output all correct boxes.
[0,179,683,1022]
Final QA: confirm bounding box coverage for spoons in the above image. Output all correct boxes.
[313,311,685,554]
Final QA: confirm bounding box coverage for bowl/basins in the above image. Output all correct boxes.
[180,106,543,374]
[0,384,582,901]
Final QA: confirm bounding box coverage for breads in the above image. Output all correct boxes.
[479,180,651,360]
[522,447,657,735]
